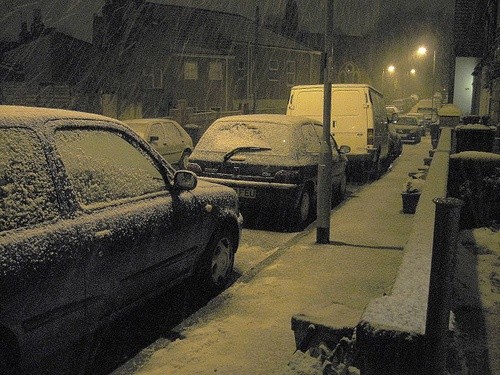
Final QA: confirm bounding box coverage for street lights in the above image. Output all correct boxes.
[419,48,435,124]
[382,65,394,98]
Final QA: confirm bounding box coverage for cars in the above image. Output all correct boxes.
[0,106,244,375]
[186,113,351,229]
[286,84,447,183]
